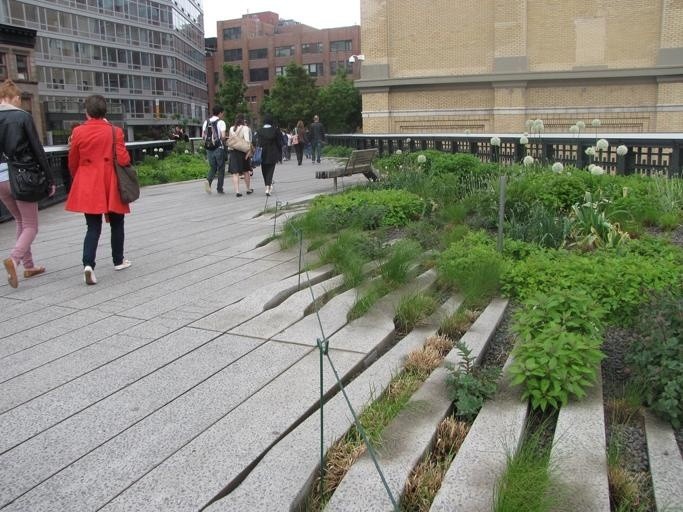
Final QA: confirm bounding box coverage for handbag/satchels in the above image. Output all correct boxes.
[292,128,299,144]
[0,149,52,202]
[227,126,254,155]
[112,126,139,203]
[272,129,283,162]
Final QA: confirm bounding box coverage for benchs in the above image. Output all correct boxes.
[315,148,375,192]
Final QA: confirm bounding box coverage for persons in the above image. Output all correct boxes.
[308,114,325,164]
[255,114,283,197]
[281,129,288,160]
[291,120,308,165]
[172,126,180,139]
[0,79,57,290]
[286,130,291,160]
[179,128,189,142]
[64,95,131,285]
[240,119,253,179]
[201,106,228,194]
[226,113,254,197]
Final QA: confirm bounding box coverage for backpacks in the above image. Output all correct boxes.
[203,118,223,151]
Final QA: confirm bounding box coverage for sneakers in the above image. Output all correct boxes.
[217,191,225,194]
[266,191,271,196]
[84,266,98,284]
[115,260,131,271]
[23,266,45,278]
[3,258,18,288]
[269,186,274,193]
[204,180,211,194]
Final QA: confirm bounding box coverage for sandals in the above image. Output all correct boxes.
[236,193,242,197]
[246,189,253,195]
